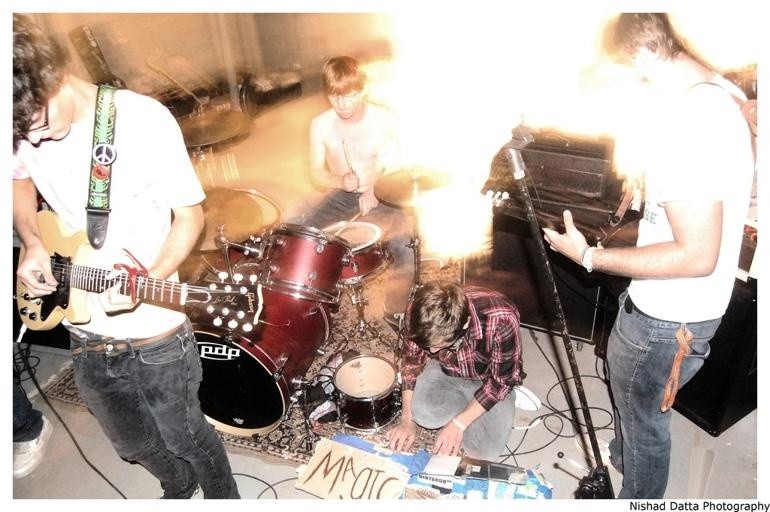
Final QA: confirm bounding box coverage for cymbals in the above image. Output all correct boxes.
[171,188,263,250]
[176,110,246,148]
[374,170,450,207]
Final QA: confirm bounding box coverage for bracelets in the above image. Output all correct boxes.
[580,240,604,273]
[453,418,466,431]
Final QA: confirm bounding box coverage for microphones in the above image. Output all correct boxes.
[510,125,536,150]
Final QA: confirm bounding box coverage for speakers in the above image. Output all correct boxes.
[490,201,640,343]
[595,254,757,436]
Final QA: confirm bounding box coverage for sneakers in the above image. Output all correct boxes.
[13,414,53,479]
[575,432,619,473]
[515,385,541,411]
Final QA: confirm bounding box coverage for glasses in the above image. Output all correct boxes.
[14,101,50,143]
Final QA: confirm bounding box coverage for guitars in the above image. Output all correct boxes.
[16,210,264,333]
[486,189,640,247]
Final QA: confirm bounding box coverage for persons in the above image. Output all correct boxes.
[13,355,54,478]
[13,12,241,498]
[299,56,414,255]
[541,12,755,500]
[385,278,527,462]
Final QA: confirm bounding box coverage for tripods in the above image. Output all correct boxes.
[325,292,395,364]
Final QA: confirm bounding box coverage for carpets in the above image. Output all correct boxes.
[25,264,464,470]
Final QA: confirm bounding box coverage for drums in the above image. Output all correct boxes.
[321,221,383,282]
[335,355,402,432]
[240,191,283,234]
[192,288,333,436]
[262,223,350,305]
